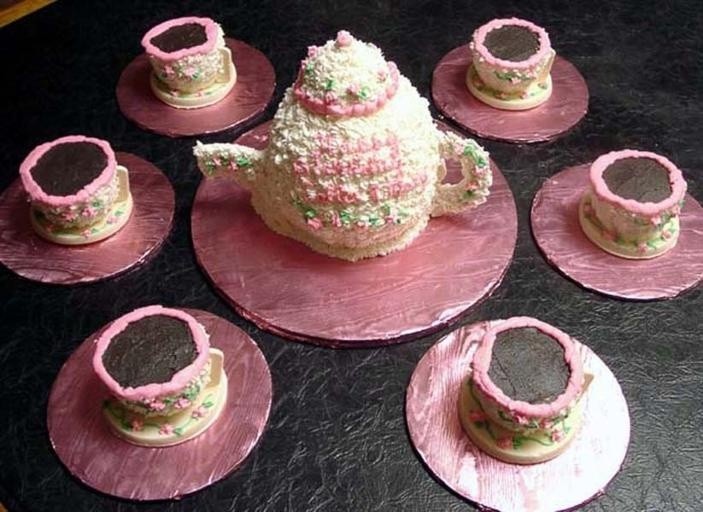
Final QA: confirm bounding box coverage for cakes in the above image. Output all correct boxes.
[91,304,229,448]
[465,16,556,113]
[16,135,135,246]
[458,314,595,462]
[190,23,495,263]
[580,147,688,260]
[141,17,239,110]
